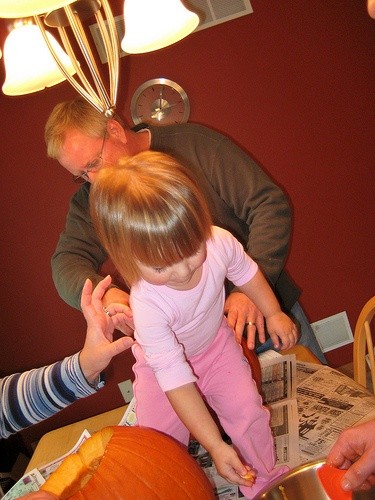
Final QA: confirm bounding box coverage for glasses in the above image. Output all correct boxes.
[71,121,109,185]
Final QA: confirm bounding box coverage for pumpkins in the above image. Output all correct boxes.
[13,426,216,500]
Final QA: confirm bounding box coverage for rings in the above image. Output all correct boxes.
[103,307,112,317]
[246,322,255,325]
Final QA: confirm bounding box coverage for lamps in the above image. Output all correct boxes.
[0,0,200,96]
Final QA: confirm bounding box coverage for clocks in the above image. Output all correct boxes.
[131,78,190,126]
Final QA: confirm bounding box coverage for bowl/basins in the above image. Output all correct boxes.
[252,458,375,500]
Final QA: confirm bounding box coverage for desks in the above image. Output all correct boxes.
[23,345,330,500]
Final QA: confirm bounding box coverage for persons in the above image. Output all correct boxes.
[44,97,329,365]
[89,150,299,500]
[327,418,375,491]
[0,276,134,500]
